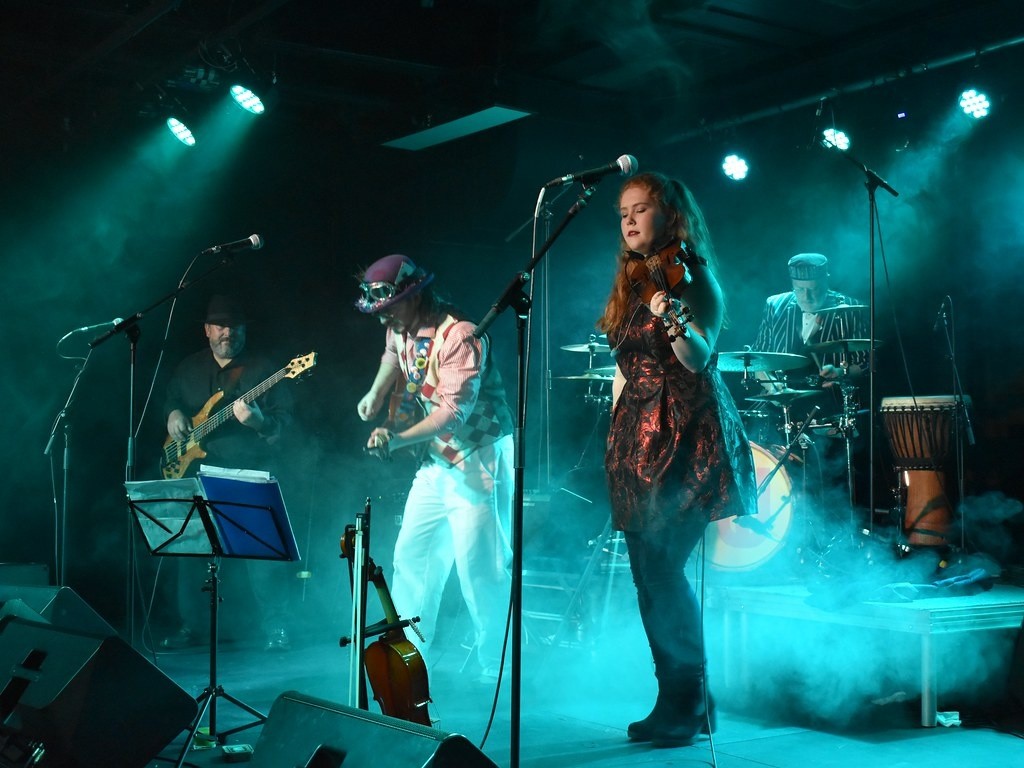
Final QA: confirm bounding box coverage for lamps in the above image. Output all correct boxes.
[146,73,199,146]
[816,98,856,154]
[955,51,993,120]
[216,40,278,115]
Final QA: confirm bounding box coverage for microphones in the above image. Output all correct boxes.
[807,99,824,150]
[72,318,124,334]
[201,234,264,255]
[544,154,638,188]
[934,296,949,331]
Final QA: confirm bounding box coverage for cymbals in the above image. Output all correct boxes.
[598,334,608,340]
[545,376,615,384]
[737,409,770,419]
[558,343,613,353]
[716,351,815,373]
[798,304,871,314]
[800,336,885,354]
[744,388,825,404]
[808,407,870,439]
[584,364,616,375]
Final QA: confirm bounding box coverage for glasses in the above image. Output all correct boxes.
[357,279,401,303]
[792,286,822,297]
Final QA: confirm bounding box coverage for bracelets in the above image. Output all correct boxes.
[662,317,670,322]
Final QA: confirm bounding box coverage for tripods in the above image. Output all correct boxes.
[126,496,293,768]
[812,341,896,566]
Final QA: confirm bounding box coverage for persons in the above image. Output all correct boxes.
[156,292,294,651]
[354,253,516,683]
[746,251,874,417]
[596,169,760,746]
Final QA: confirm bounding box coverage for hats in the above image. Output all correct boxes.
[354,254,434,312]
[201,292,253,327]
[787,253,829,281]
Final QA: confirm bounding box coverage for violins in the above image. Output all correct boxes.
[630,236,695,343]
[338,494,434,731]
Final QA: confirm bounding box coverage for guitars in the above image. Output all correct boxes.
[158,350,320,479]
[362,372,425,462]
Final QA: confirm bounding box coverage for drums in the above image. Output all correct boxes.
[690,438,796,572]
[879,395,973,548]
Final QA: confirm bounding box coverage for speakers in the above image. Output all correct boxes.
[246,691,501,768]
[0,560,198,768]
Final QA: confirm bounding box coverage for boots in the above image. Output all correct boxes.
[627,661,674,743]
[646,655,717,749]
